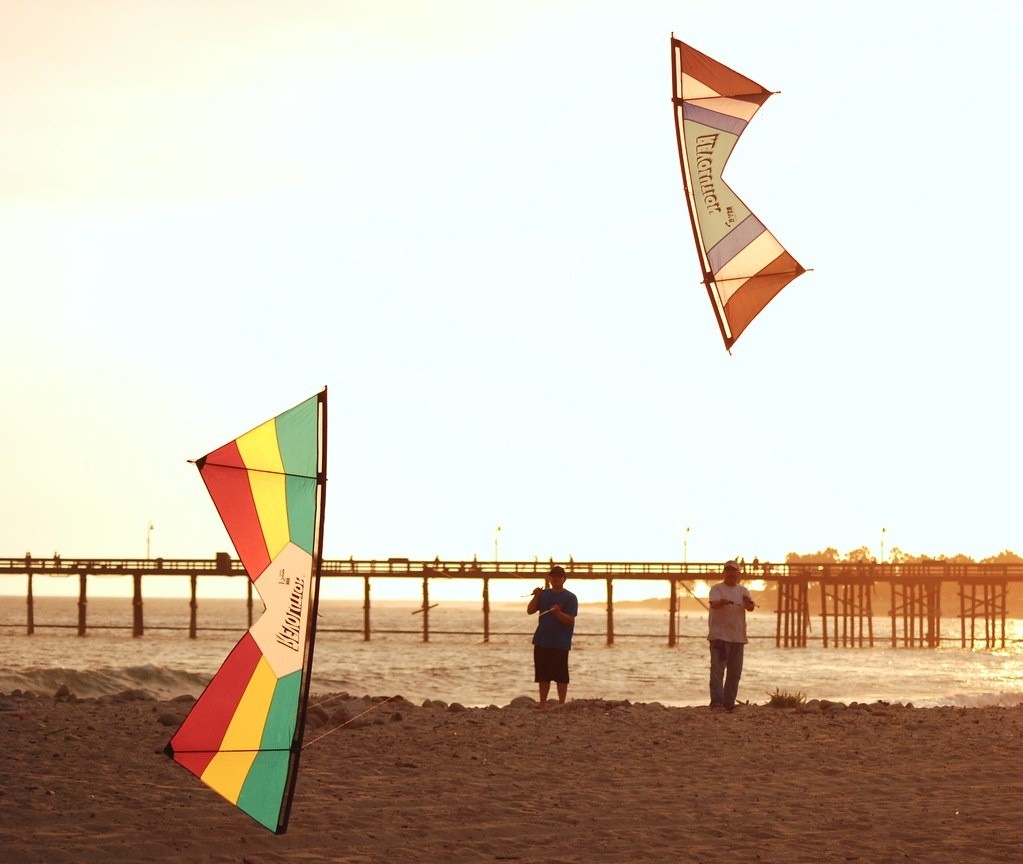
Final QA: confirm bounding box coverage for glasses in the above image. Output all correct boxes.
[551,574,564,578]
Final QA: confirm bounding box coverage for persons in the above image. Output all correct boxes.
[527,566,578,709]
[707,560,755,711]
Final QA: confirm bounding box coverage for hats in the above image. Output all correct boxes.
[549,566,565,575]
[724,561,739,569]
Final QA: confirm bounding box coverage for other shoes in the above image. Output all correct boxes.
[713,706,722,713]
[725,707,744,714]
